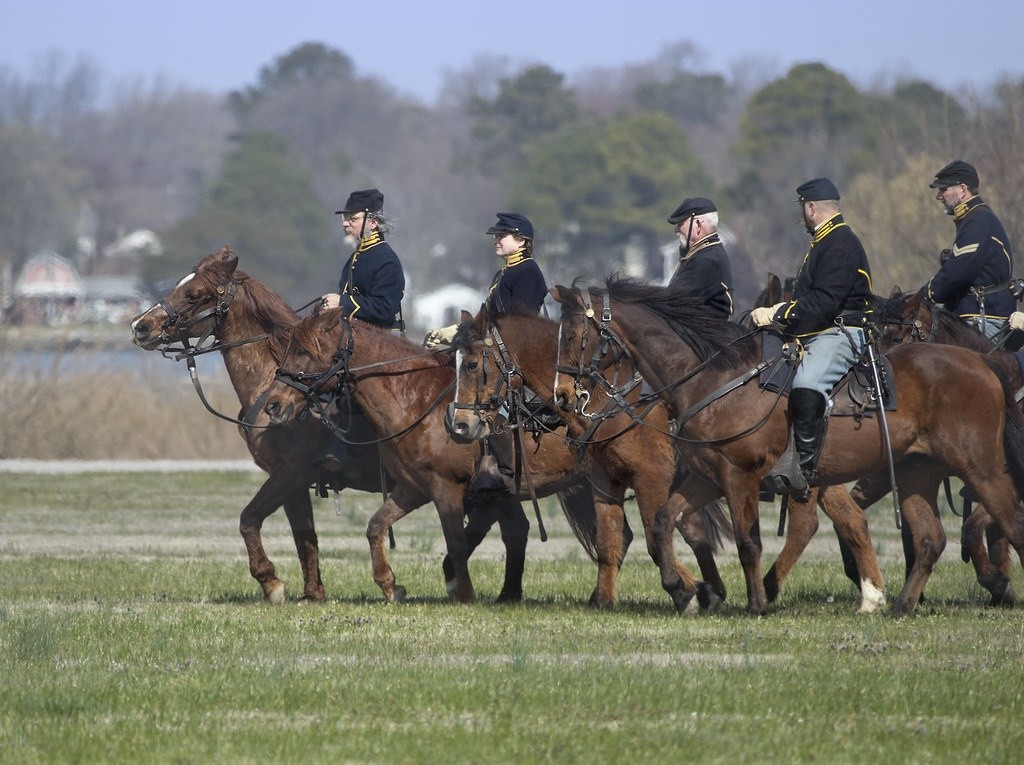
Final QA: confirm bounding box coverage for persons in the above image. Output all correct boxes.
[424,213,550,368]
[749,177,874,502]
[319,189,406,331]
[924,159,1018,352]
[664,197,736,320]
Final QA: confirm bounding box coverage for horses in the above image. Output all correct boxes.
[128,246,1023,616]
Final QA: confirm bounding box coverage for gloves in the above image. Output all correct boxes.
[1009,311,1024,330]
[427,323,459,347]
[750,302,785,327]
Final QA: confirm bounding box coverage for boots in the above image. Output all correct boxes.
[488,413,515,494]
[766,387,826,502]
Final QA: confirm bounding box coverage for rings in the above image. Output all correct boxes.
[323,304,326,307]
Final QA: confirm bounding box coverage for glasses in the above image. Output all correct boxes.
[491,233,511,240]
[340,214,374,224]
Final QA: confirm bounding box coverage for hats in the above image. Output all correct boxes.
[667,198,716,223]
[336,188,385,214]
[929,160,979,190]
[792,178,840,202]
[486,213,535,239]
